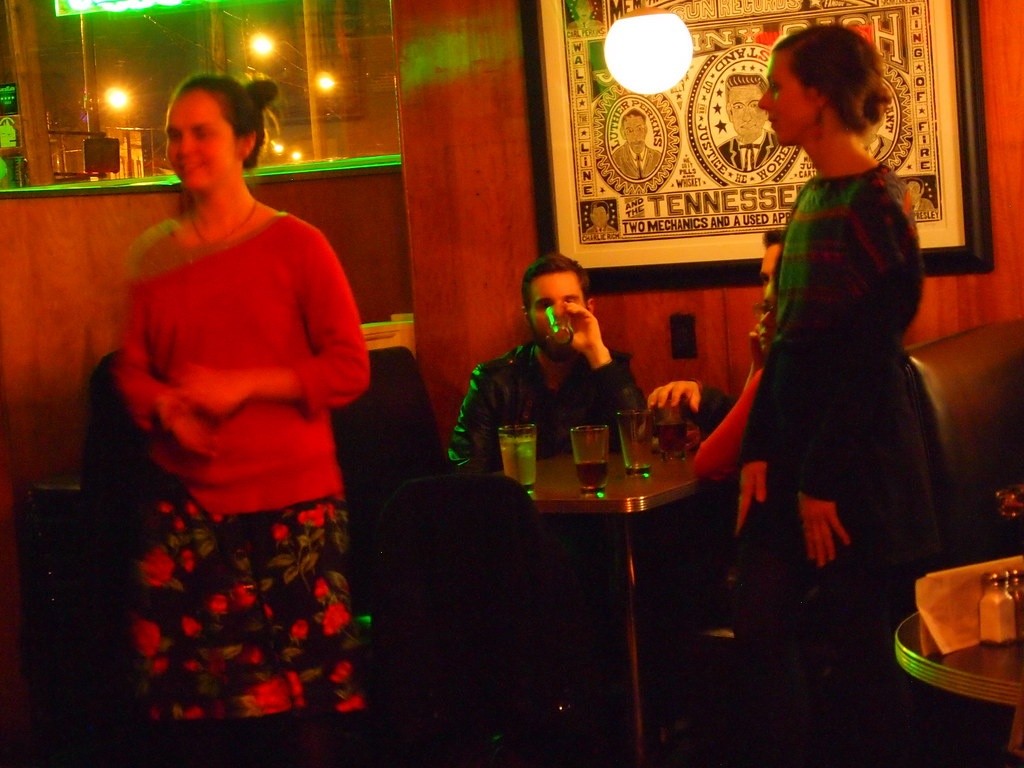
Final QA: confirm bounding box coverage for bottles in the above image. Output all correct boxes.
[1009,569,1024,644]
[980,571,1015,646]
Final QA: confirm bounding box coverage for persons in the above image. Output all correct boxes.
[647,227,790,432]
[114,71,374,767]
[447,252,650,476]
[692,278,781,481]
[734,25,946,768]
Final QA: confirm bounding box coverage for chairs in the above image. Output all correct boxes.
[904,320,1023,557]
[372,473,587,768]
[332,344,443,625]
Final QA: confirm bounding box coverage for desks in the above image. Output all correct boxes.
[493,442,704,768]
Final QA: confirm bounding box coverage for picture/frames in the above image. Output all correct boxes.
[518,0,995,294]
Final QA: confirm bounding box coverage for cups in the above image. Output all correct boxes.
[569,425,610,493]
[545,304,573,344]
[655,400,688,461]
[497,423,536,494]
[617,410,654,477]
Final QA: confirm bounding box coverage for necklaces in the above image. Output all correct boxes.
[189,199,258,247]
